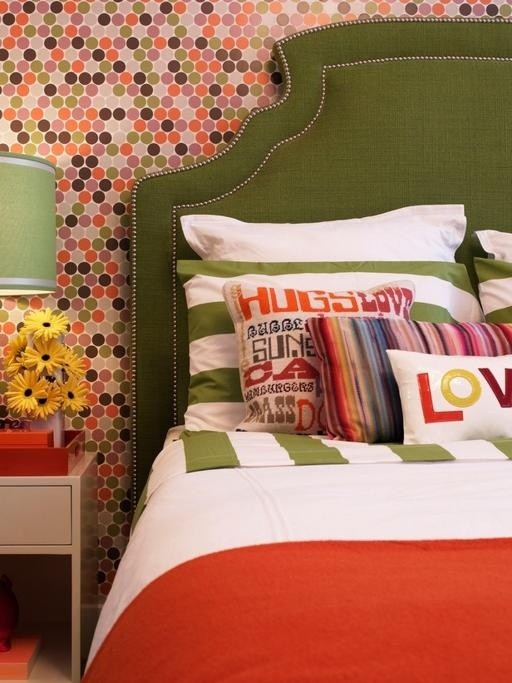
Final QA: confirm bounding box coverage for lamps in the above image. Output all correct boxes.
[0,144,62,298]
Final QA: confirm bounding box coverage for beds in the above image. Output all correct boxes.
[78,8,512,677]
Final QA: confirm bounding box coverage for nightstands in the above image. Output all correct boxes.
[0,448,114,683]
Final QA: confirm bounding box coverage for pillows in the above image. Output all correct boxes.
[176,258,485,441]
[472,228,512,320]
[181,202,469,263]
[222,279,417,439]
[385,349,511,448]
[307,317,512,444]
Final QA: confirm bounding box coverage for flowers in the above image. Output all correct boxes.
[2,305,92,420]
